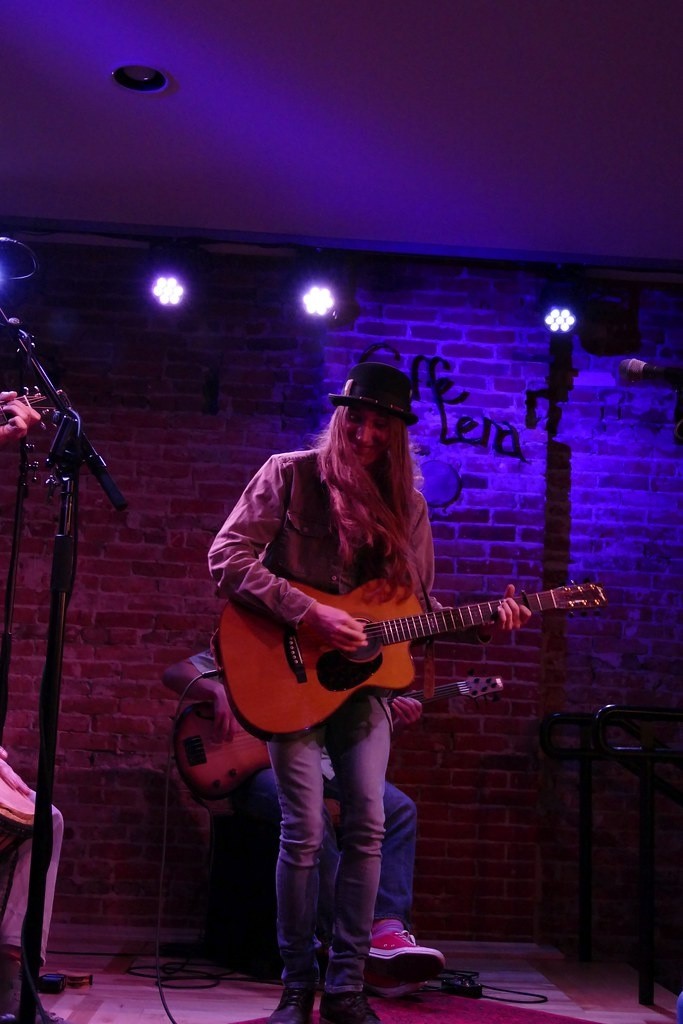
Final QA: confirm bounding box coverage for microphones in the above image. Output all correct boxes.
[202,670,220,680]
[617,355,682,389]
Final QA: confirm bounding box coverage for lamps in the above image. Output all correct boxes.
[151,273,186,306]
[302,283,338,315]
[543,305,578,335]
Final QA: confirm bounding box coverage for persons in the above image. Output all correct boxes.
[159,627,445,995]
[0,391,43,445]
[206,363,534,1024]
[0,744,64,1024]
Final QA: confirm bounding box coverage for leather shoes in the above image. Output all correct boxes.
[271,989,314,1024]
[318,993,385,1024]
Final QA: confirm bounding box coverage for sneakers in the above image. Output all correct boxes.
[359,928,445,996]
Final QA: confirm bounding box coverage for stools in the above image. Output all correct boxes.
[175,774,342,967]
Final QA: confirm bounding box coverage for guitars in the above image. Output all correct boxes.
[216,577,608,739]
[1,384,72,432]
[173,666,503,800]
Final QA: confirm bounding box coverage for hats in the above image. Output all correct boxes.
[329,363,421,424]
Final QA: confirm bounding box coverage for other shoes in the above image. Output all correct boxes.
[3,966,65,1023]
